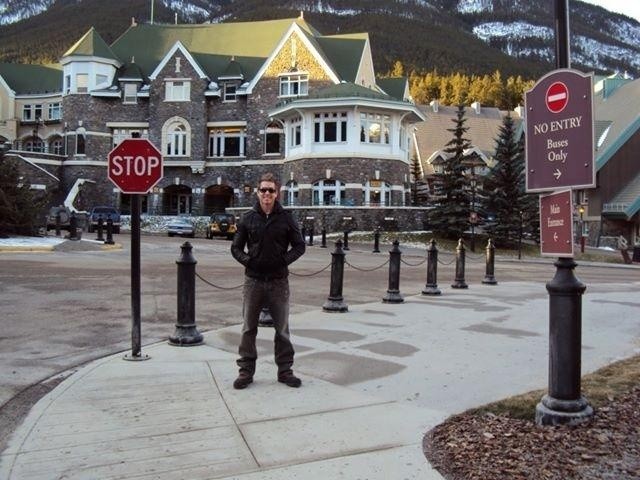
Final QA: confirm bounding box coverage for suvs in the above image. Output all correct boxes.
[206,214,237,239]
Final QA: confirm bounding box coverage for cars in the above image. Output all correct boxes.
[88,205,122,233]
[45,207,72,231]
[168,219,195,237]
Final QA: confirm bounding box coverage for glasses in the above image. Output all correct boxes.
[258,186,277,195]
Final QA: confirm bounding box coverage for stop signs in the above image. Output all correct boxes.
[107,138,164,195]
[544,79,570,114]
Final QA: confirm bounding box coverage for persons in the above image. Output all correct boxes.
[230,171,306,390]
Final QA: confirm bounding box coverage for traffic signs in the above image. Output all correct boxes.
[540,188,573,259]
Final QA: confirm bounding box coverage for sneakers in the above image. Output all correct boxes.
[233,371,254,390]
[278,375,301,388]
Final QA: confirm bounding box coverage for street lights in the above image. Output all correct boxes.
[579,208,586,253]
